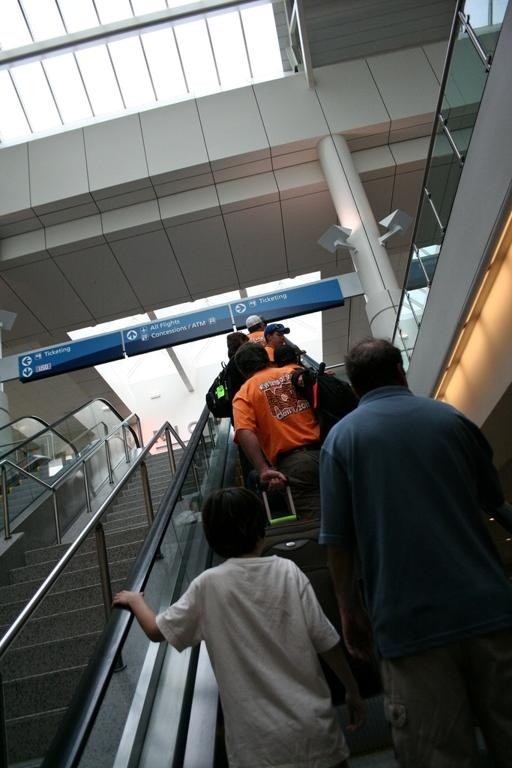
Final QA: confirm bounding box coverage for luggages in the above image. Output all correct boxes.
[259,475,362,663]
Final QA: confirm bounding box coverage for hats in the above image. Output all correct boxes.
[245,314,262,328]
[265,324,290,336]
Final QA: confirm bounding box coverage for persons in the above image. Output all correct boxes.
[231,341,325,522]
[109,484,358,767]
[270,341,336,376]
[260,323,290,363]
[319,333,512,767]
[244,314,268,342]
[219,332,272,495]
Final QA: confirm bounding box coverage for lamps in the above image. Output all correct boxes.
[0,309,17,331]
[318,225,359,255]
[377,208,413,247]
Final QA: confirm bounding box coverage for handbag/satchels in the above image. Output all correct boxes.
[206,391,230,417]
[302,369,357,443]
